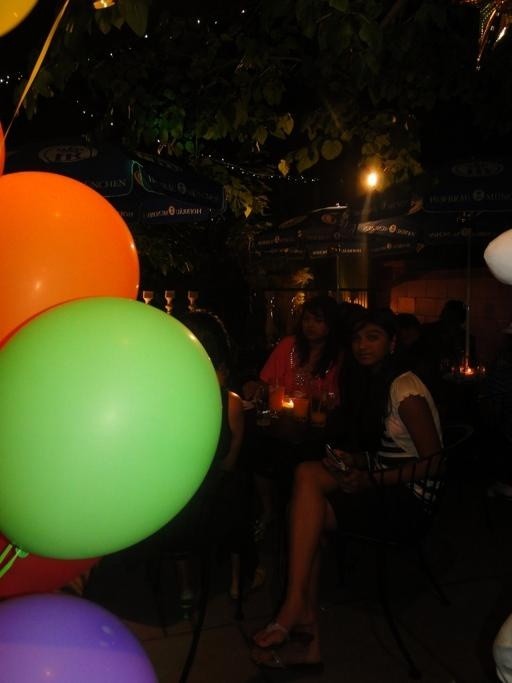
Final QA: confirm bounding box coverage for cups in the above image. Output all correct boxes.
[253,369,331,428]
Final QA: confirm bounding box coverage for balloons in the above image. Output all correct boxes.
[0,128,223,683]
[1,0,37,37]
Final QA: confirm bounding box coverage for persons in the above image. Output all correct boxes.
[223,298,512,682]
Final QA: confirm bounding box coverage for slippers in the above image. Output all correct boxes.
[251,621,322,667]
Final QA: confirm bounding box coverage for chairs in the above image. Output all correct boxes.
[147,466,287,682]
[318,425,466,679]
